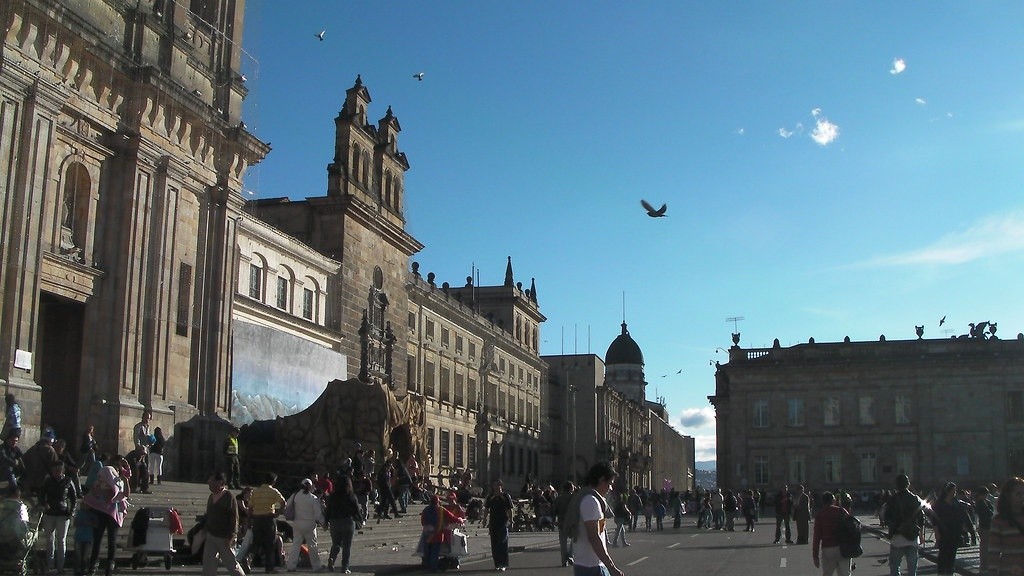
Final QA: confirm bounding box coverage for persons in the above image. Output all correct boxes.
[610,480,763,551]
[985,475,1023,576]
[774,484,795,545]
[574,461,625,576]
[831,487,999,548]
[791,485,811,544]
[975,487,994,574]
[4,393,485,576]
[886,473,924,576]
[521,478,557,529]
[932,482,966,576]
[551,480,578,566]
[485,480,514,573]
[812,492,853,576]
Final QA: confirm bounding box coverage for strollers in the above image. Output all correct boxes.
[1,509,45,575]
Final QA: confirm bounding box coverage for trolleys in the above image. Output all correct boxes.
[123,506,177,570]
[413,519,467,571]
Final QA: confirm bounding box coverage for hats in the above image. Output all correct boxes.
[446,492,457,500]
[301,478,313,486]
[430,495,441,502]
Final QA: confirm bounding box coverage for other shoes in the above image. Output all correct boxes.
[328,557,335,571]
[342,569,352,574]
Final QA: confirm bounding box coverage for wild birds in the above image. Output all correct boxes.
[413,72,424,81]
[940,316,946,326]
[640,200,667,217]
[661,375,667,377]
[676,369,682,374]
[314,29,326,41]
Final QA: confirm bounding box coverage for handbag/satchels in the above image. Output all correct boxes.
[839,506,864,559]
[284,488,300,521]
